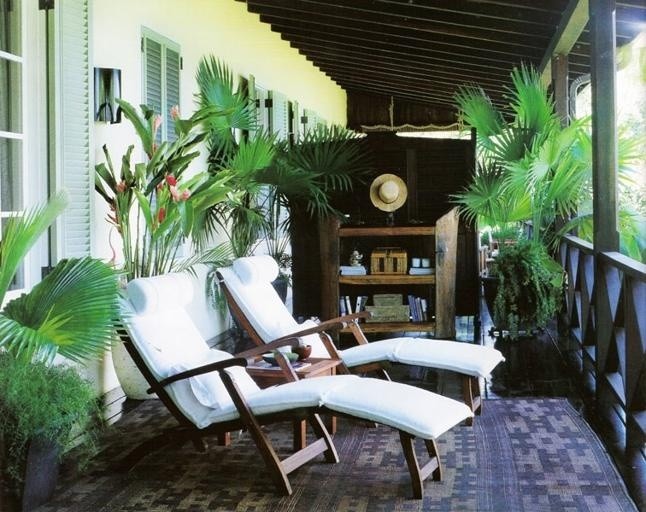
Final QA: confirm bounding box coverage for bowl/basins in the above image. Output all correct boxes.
[262,352,300,364]
[292,345,311,361]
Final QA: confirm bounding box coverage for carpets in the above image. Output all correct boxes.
[34,395,641,512]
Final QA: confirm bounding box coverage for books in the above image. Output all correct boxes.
[340,296,368,328]
[245,356,312,372]
[340,266,365,271]
[408,295,428,323]
[341,270,367,276]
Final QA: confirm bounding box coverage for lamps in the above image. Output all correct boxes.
[94,67,121,126]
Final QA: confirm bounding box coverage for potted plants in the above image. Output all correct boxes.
[194,48,370,339]
[445,58,646,335]
[0,192,127,512]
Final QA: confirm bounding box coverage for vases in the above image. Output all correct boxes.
[110,327,159,399]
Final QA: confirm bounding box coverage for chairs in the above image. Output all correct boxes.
[110,274,473,497]
[215,255,507,428]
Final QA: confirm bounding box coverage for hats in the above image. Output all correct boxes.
[368,172,410,212]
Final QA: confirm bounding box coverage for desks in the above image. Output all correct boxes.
[217,354,343,451]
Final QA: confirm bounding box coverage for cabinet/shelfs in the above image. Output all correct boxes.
[320,205,460,349]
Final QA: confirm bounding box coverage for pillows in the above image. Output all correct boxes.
[167,348,261,428]
[286,319,334,359]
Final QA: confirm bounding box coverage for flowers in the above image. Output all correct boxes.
[90,96,246,282]
